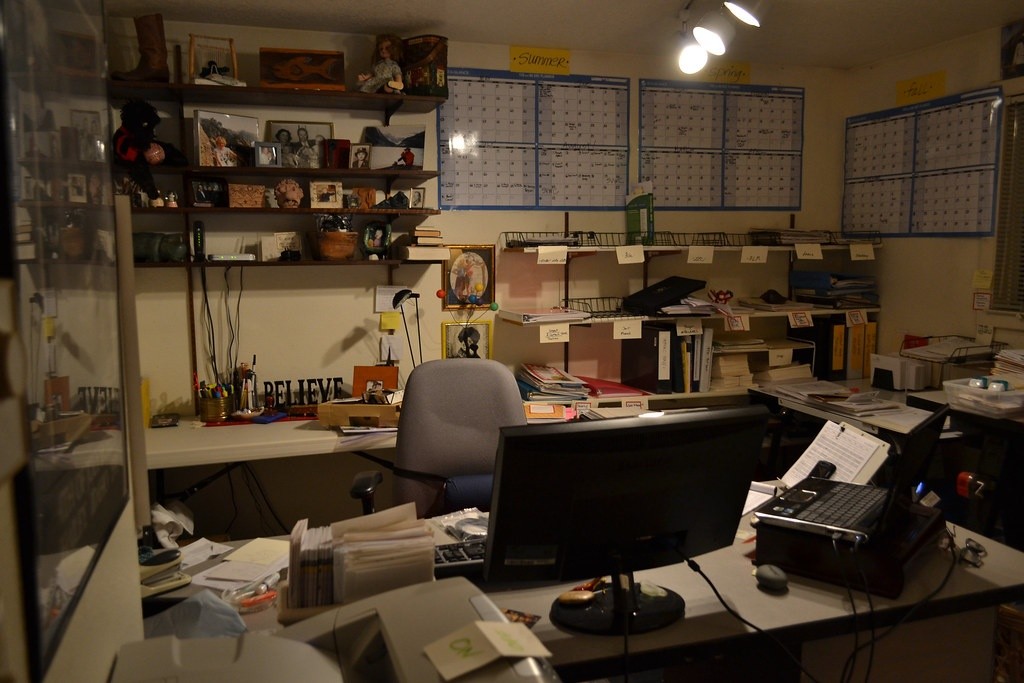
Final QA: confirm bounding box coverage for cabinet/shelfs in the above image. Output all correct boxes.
[108,79,445,268]
[5,68,107,267]
[499,229,883,327]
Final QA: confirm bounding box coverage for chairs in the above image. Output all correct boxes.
[349,359,527,520]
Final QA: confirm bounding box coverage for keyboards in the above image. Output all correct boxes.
[434,539,487,581]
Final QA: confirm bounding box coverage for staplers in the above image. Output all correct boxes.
[139,548,192,599]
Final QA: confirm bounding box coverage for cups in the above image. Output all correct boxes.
[200,398,232,421]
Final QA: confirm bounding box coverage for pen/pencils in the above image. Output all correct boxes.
[194,373,234,398]
[257,572,280,594]
[234,354,256,410]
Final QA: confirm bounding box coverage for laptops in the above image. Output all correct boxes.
[755,401,952,545]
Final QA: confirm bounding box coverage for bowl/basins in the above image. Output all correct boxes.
[305,231,359,260]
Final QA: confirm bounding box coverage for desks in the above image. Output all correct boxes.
[778,378,1024,446]
[181,477,1024,682]
[145,389,747,469]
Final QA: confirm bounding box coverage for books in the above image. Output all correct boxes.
[516,360,590,400]
[289,501,438,608]
[621,324,813,395]
[789,271,882,310]
[399,226,451,260]
[768,379,905,419]
[989,349,1024,378]
[787,315,876,380]
[749,227,832,245]
[869,337,988,390]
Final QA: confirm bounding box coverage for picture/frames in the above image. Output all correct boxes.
[349,144,372,169]
[267,120,335,169]
[363,222,392,253]
[20,27,105,202]
[183,174,229,207]
[441,244,496,311]
[309,181,344,209]
[409,187,425,209]
[441,322,492,360]
[255,142,282,167]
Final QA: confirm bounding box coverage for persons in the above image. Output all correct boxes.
[355,31,409,95]
[392,147,415,169]
[274,129,296,154]
[294,127,315,153]
[352,147,369,168]
[260,147,277,166]
[213,137,238,167]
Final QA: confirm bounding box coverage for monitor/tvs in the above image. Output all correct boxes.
[486,404,772,637]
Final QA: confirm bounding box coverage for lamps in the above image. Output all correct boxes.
[392,289,423,370]
[693,0,777,57]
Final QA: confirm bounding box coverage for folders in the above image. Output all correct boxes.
[513,377,587,402]
[623,276,713,317]
[620,324,675,395]
[498,305,590,323]
[574,375,652,397]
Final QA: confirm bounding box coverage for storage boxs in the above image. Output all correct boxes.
[942,378,1024,420]
